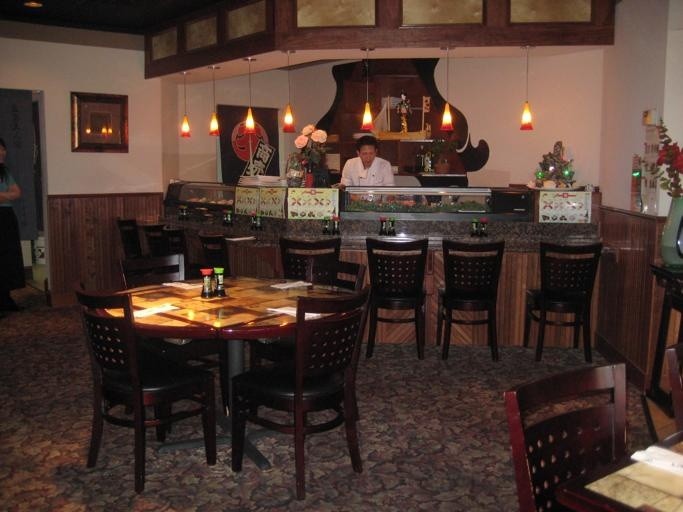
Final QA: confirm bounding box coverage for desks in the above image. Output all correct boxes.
[558,431,682,512]
[117,280,350,462]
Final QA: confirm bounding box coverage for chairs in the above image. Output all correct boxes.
[227,284,368,500]
[70,290,224,489]
[142,223,167,276]
[198,230,231,274]
[665,344,682,443]
[364,237,428,360]
[161,224,201,276]
[289,262,368,421]
[435,239,506,361]
[522,242,604,361]
[503,364,628,512]
[118,253,233,431]
[279,237,341,283]
[117,218,153,279]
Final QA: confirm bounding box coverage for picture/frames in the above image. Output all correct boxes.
[70,92,130,154]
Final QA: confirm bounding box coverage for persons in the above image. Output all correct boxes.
[331,135,395,189]
[396,88,411,134]
[0,137,27,315]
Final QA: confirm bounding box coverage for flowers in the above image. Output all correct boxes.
[650,118,682,197]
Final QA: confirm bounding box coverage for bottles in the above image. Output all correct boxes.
[249,209,264,230]
[199,268,215,298]
[321,214,340,234]
[213,267,226,299]
[470,217,489,239]
[223,209,233,227]
[378,215,397,236]
[178,204,189,220]
[286,152,304,188]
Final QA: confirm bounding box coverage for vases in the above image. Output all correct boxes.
[658,197,682,267]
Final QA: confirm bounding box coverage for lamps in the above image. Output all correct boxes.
[283,47,297,135]
[207,64,220,137]
[361,48,376,131]
[243,56,256,136]
[520,47,535,134]
[178,70,191,136]
[440,47,453,133]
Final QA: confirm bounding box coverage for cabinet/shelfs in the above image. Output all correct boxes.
[642,261,682,416]
